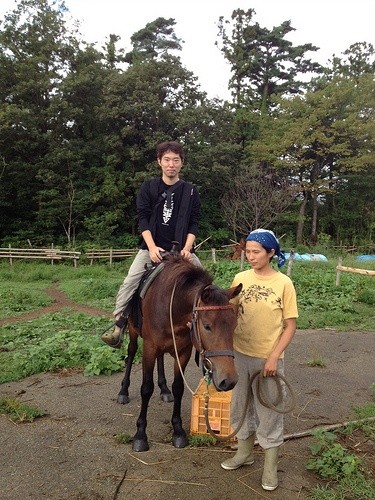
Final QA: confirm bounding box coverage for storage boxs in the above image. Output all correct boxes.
[190,376,237,442]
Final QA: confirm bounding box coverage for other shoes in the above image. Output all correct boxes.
[102,332,123,350]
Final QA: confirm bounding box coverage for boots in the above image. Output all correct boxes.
[220,433,256,470]
[262,447,280,490]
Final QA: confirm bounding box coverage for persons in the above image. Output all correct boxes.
[220,228,298,491]
[101,139,207,349]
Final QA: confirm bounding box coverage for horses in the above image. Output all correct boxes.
[116,252,243,452]
